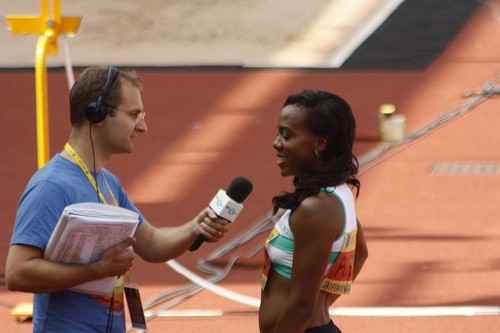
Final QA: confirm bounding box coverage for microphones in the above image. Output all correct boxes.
[188,176,253,252]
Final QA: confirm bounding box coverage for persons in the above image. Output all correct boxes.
[258,89,368,333]
[5,66,228,333]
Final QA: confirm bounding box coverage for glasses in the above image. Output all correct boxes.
[106,104,147,120]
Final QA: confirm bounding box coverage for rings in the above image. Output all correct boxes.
[218,232,221,238]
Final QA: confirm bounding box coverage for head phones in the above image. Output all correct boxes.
[85,66,119,124]
